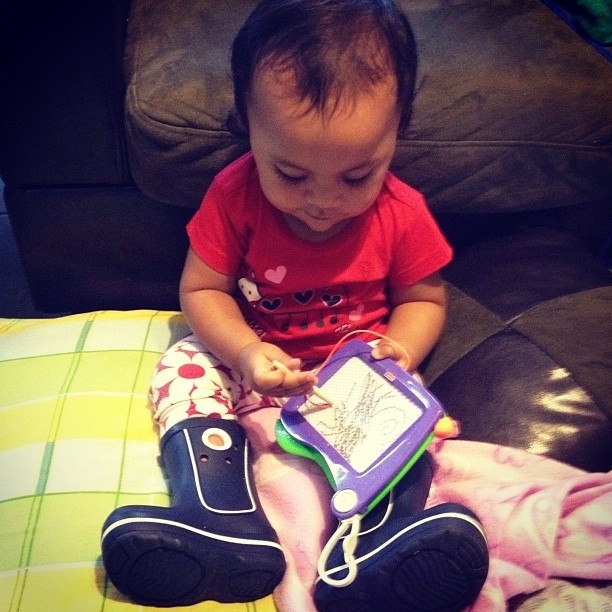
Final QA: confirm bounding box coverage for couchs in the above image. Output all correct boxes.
[0,0,612,471]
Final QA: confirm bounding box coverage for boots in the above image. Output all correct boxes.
[100,417,287,606]
[311,449,488,610]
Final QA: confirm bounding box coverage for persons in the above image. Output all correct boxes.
[100,0,490,611]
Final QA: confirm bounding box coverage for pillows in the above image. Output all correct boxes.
[125,3,606,208]
[0,308,275,612]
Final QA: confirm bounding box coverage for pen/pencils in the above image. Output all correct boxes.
[273,361,334,406]
